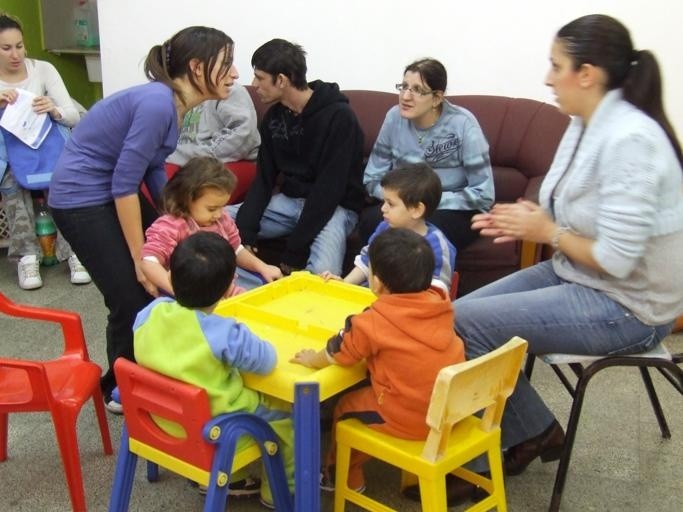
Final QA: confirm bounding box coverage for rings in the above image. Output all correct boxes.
[507,219,511,226]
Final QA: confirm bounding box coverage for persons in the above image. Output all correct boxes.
[290,229,466,497]
[129,231,290,507]
[137,158,283,299]
[141,81,259,212]
[0,12,94,291]
[318,163,456,298]
[400,16,682,506]
[363,58,495,251]
[45,26,238,414]
[224,37,362,291]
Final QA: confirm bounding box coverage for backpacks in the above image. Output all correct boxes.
[0,106,71,195]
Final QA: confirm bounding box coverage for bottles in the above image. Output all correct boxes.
[31,197,60,266]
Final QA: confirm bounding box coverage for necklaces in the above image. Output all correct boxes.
[415,111,437,146]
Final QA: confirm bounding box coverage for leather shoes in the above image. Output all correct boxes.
[402,472,490,507]
[504,419,565,476]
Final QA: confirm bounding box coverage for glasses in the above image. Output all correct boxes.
[395,84,436,95]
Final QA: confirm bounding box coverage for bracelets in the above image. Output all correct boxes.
[551,227,567,248]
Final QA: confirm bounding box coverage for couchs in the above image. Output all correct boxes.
[243,84,572,296]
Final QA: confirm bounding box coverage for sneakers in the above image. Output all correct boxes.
[102,393,123,414]
[198,476,261,496]
[319,463,366,494]
[67,254,91,284]
[16,254,42,289]
